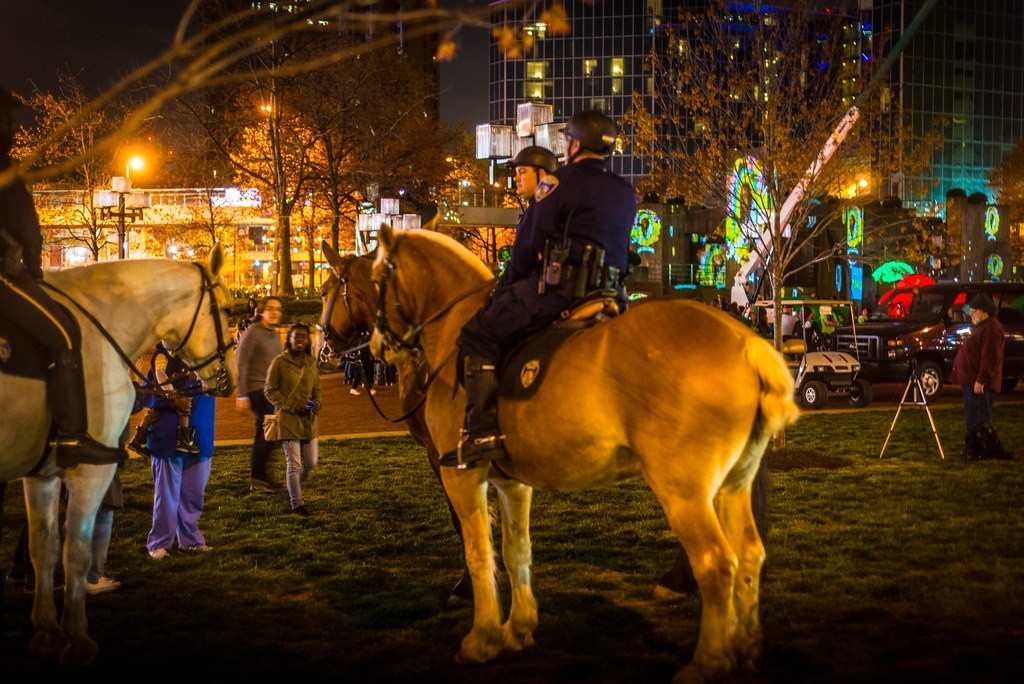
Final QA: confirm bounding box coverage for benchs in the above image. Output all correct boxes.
[762,335,808,368]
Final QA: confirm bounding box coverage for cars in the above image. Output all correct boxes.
[749,297,874,409]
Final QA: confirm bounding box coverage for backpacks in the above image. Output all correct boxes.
[965,418,1016,460]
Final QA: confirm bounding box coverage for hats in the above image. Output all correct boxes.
[783,305,793,308]
[970,293,997,317]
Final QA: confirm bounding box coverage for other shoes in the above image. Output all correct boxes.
[343,379,395,386]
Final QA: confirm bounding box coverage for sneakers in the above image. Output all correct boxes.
[149,548,170,561]
[350,389,361,395]
[251,470,269,483]
[371,389,376,395]
[178,544,213,553]
[6,562,35,582]
[23,576,64,595]
[294,506,310,517]
[87,577,120,594]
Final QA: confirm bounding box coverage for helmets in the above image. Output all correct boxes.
[0,87,22,108]
[559,110,616,154]
[507,146,558,173]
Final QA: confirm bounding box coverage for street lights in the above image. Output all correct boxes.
[477,102,572,202]
[92,176,152,263]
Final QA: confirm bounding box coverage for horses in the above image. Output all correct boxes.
[311,220,803,684]
[0,242,239,677]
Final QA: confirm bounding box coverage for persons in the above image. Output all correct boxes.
[243,298,257,328]
[948,293,1005,462]
[132,342,215,562]
[694,294,869,353]
[235,296,284,494]
[263,323,323,518]
[6,465,124,597]
[0,87,130,468]
[342,333,397,396]
[440,112,637,467]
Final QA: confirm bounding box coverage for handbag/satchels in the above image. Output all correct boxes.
[264,415,278,440]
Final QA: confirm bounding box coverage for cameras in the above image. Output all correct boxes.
[307,402,314,408]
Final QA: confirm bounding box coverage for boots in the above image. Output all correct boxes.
[178,427,200,454]
[128,425,150,458]
[440,355,509,467]
[48,349,128,468]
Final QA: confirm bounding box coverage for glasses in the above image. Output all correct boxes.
[265,306,285,311]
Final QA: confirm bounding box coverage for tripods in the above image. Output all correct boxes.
[878,368,945,459]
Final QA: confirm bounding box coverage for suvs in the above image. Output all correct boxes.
[827,280,1024,404]
